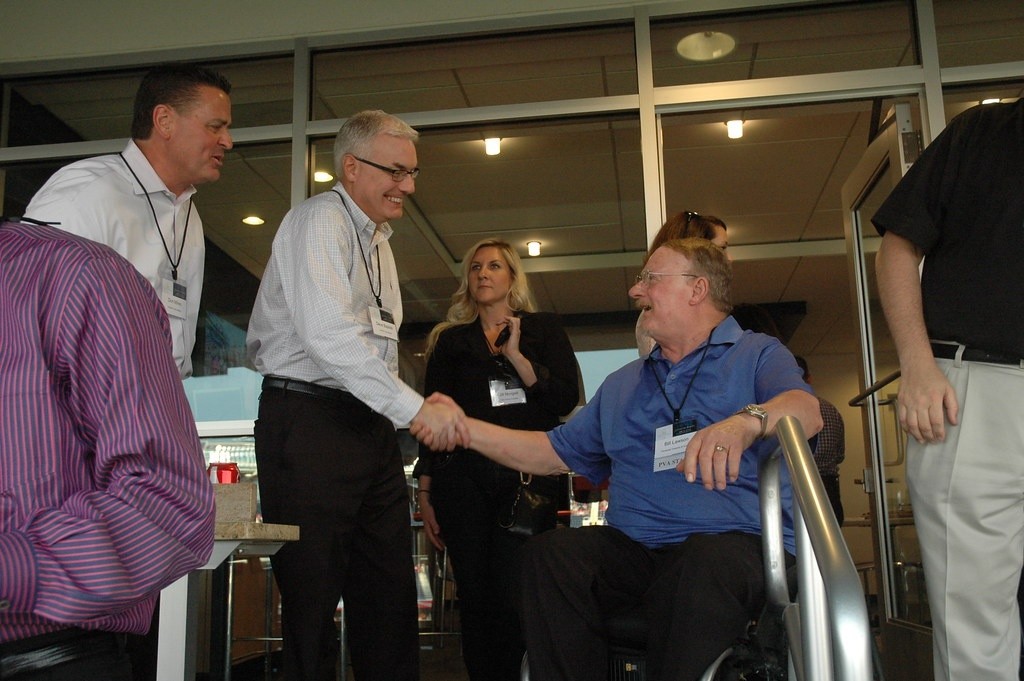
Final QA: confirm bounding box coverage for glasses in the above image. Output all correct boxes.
[683,210,698,239]
[634,270,700,286]
[354,155,421,182]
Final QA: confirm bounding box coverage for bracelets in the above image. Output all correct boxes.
[416,489,432,495]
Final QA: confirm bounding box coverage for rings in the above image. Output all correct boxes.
[714,445,728,453]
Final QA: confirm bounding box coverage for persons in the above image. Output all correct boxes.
[869,98,1024,681]
[406,237,826,681]
[793,355,846,528]
[18,60,234,381]
[246,107,472,681]
[0,218,217,681]
[635,210,734,358]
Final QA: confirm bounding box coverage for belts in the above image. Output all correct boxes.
[0,629,130,677]
[929,340,1023,366]
[261,377,377,412]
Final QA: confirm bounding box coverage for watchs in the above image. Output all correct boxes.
[732,404,768,440]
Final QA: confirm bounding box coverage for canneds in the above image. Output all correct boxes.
[206,462,240,484]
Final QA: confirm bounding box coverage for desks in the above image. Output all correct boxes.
[844,513,916,610]
[208,523,300,681]
